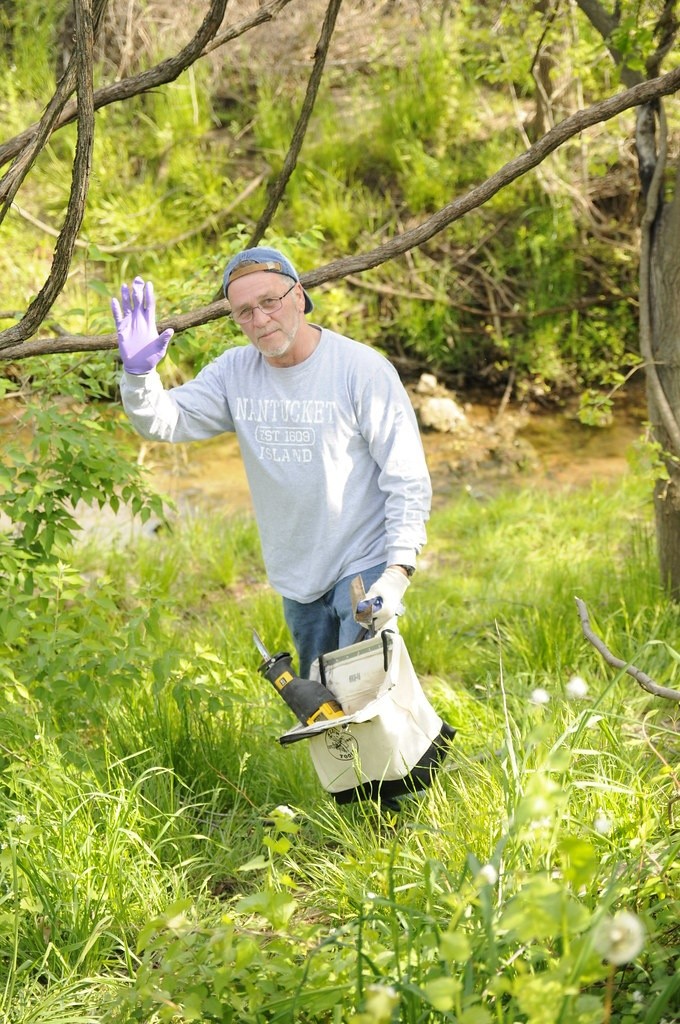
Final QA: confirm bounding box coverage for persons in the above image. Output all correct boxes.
[112,246,433,827]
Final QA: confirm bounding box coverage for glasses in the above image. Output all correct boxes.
[228,286,299,325]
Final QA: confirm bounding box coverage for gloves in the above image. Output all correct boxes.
[110,276,175,375]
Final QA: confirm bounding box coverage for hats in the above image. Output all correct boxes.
[223,248,313,315]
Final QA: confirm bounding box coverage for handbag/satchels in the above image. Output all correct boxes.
[282,631,455,805]
